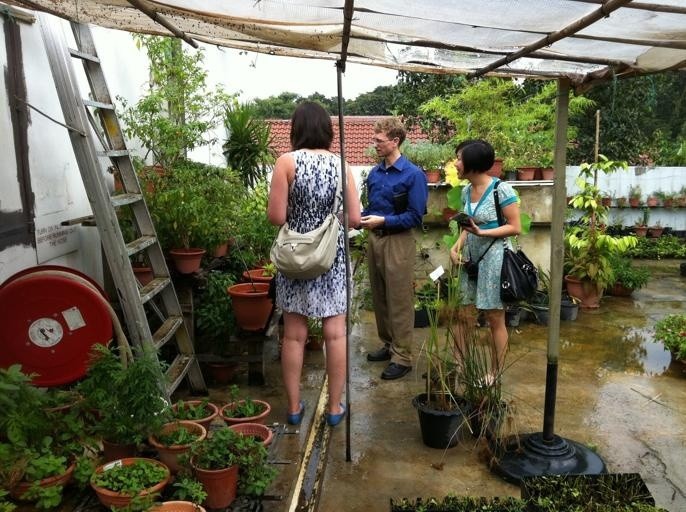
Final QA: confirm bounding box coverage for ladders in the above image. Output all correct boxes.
[39,11,210,407]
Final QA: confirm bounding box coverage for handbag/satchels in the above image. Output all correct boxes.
[499,248,537,302]
[392,192,427,215]
[464,259,478,280]
[268,212,338,280]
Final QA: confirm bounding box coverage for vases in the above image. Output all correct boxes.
[671,348,686,366]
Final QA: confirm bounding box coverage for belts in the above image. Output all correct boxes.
[371,227,411,237]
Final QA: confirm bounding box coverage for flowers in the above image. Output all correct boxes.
[650,312,686,361]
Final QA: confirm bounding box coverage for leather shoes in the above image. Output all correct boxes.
[287,400,303,425]
[367,348,390,362]
[380,363,411,380]
[327,403,345,427]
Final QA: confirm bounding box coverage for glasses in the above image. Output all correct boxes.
[370,137,393,143]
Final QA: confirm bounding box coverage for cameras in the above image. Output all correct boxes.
[463,261,479,281]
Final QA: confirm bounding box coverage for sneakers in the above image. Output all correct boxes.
[473,373,502,387]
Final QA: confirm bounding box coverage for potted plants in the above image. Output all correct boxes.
[0,338,282,511]
[111,156,324,384]
[365,133,557,182]
[412,241,536,466]
[533,151,686,326]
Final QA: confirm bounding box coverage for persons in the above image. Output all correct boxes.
[267,101,362,427]
[450,139,521,388]
[354,118,428,380]
